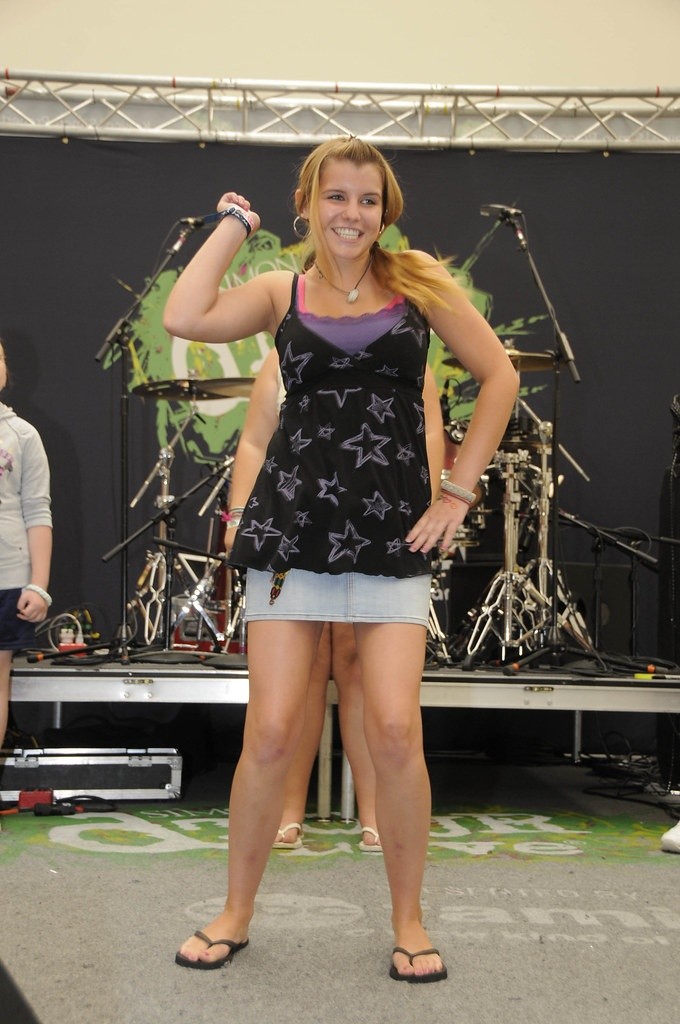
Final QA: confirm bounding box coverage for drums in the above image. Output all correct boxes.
[442,417,488,509]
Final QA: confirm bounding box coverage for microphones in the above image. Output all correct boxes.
[178,216,214,228]
[440,380,449,426]
[480,204,523,220]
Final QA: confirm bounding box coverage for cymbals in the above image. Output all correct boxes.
[195,376,257,399]
[132,375,230,402]
[443,329,535,352]
[440,347,556,372]
[498,439,552,449]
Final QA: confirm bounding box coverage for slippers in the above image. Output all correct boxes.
[175,930,250,969]
[359,826,383,851]
[271,822,304,849]
[389,947,447,983]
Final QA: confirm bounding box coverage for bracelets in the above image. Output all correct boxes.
[26,584,52,607]
[227,519,240,528]
[227,508,244,517]
[221,208,253,236]
[441,480,475,503]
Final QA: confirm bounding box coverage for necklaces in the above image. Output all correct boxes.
[314,258,371,303]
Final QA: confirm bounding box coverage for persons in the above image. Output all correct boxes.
[164,136,520,980]
[0,346,52,751]
[224,253,446,851]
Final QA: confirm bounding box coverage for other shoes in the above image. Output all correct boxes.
[660,819,680,852]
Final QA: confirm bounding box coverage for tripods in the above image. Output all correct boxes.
[456,216,680,679]
[26,227,252,668]
